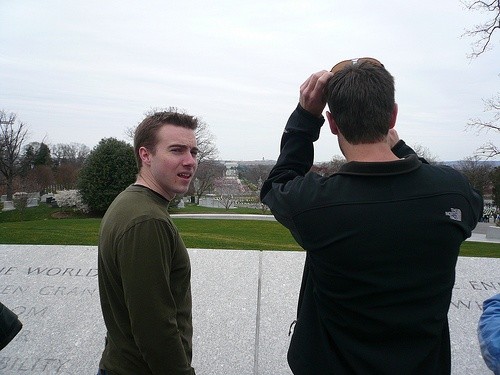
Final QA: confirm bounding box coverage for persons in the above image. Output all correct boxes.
[477,294,500,375]
[259,61,483,375]
[98,111,200,375]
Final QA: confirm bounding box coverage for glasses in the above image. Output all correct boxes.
[329,58,385,73]
